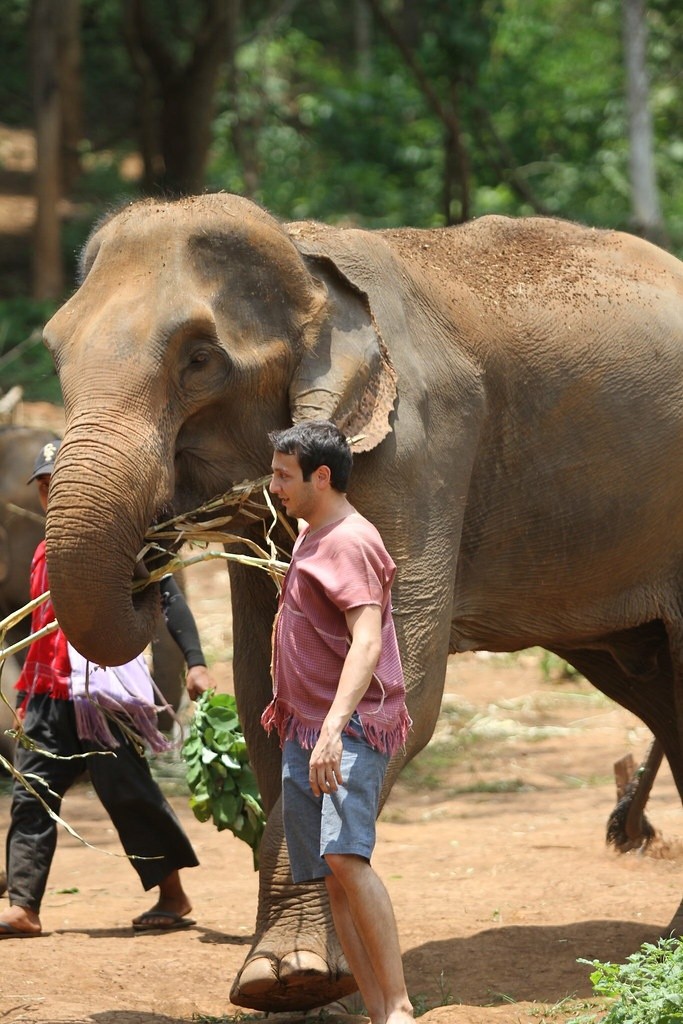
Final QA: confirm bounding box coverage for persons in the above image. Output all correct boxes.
[1,440,217,935]
[260,423,414,1024]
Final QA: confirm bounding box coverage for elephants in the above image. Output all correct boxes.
[0,192,683,1015]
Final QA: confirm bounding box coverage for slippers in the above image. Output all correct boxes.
[132,911,197,930]
[0,921,42,939]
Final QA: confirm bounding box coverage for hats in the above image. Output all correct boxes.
[26,440,62,485]
